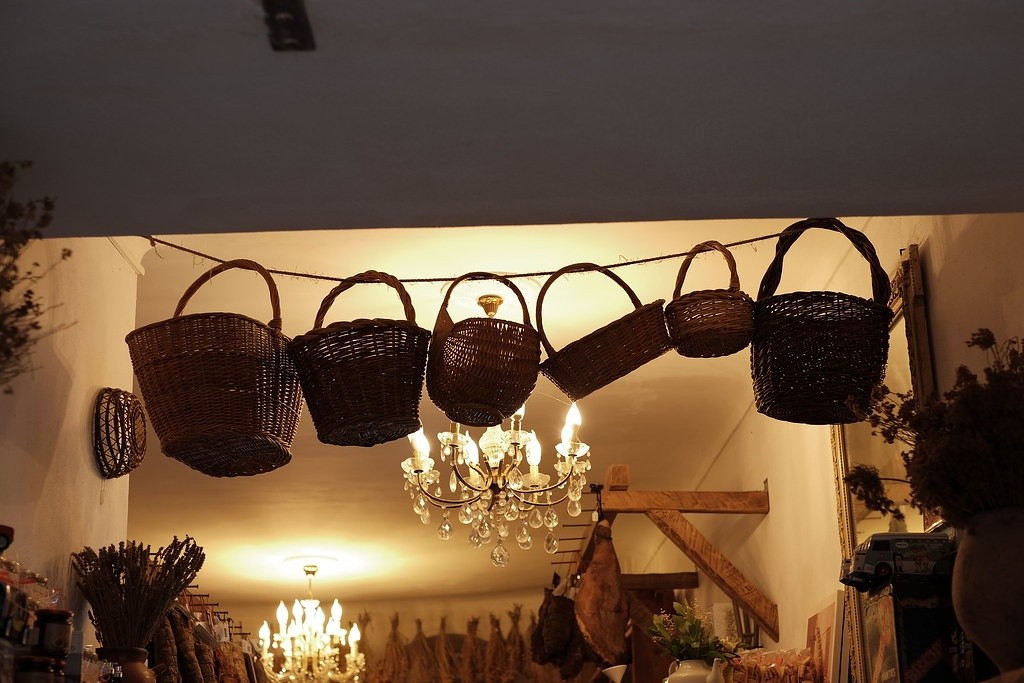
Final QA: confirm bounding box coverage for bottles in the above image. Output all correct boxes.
[83,650,123,683]
[0,556,55,646]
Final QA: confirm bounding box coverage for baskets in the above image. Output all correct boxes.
[664,240,752,358]
[93,387,147,479]
[125,259,305,479]
[286,270,431,448]
[535,263,674,402]
[426,272,541,427]
[751,217,895,425]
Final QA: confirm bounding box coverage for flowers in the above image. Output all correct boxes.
[647,598,747,671]
[71,533,205,648]
[841,324,1024,528]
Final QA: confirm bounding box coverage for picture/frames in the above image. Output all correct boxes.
[826,246,946,683]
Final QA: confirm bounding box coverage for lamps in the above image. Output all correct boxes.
[401,293,591,568]
[255,563,367,683]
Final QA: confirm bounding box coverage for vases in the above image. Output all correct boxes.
[94,647,156,683]
[666,658,725,683]
[950,507,1024,675]
